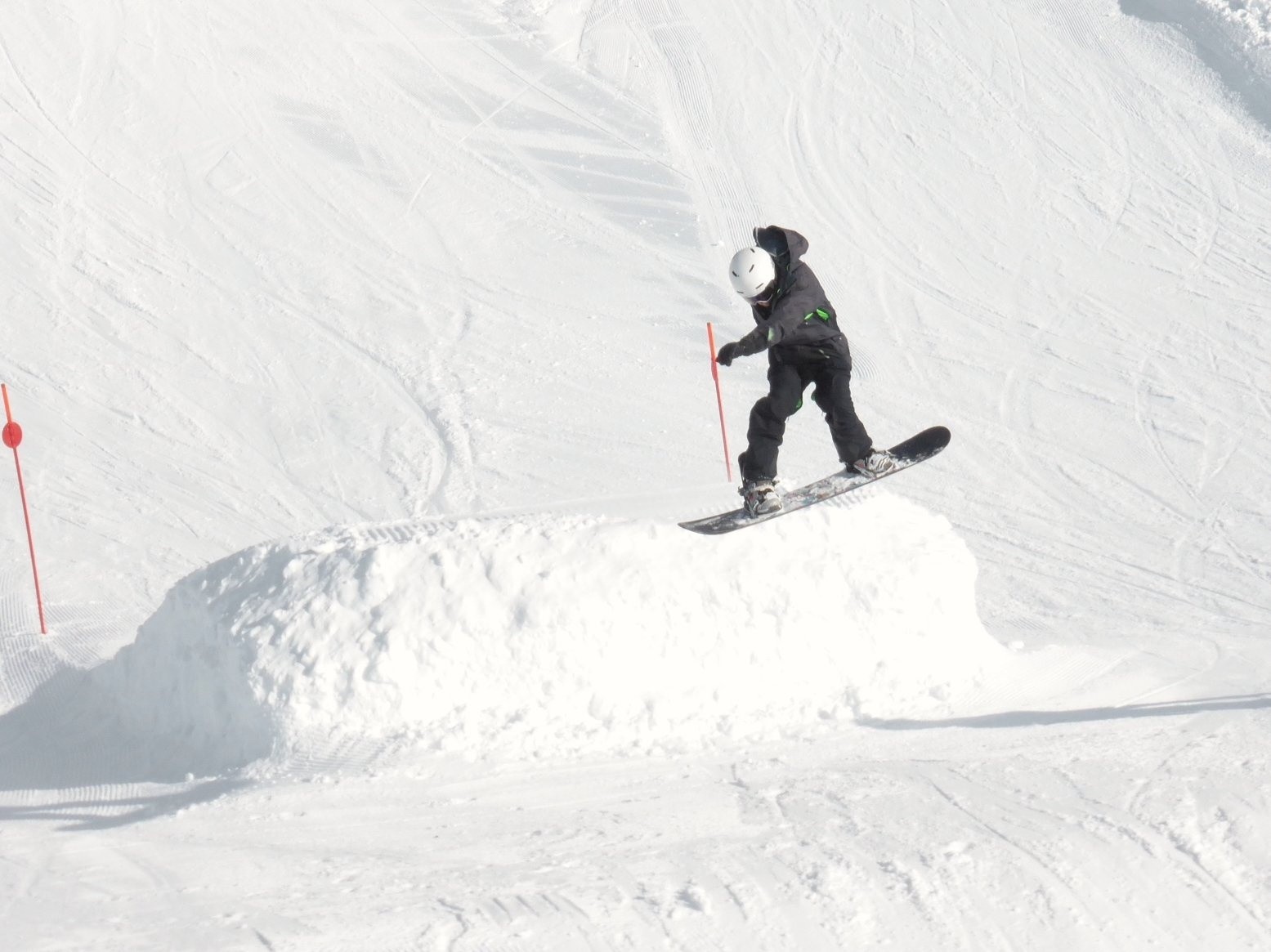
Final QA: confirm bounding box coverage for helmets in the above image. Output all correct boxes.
[730,246,777,308]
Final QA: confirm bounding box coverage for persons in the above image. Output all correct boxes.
[717,226,895,516]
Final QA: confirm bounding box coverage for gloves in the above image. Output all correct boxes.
[715,342,746,366]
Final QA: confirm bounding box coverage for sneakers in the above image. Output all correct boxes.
[845,450,894,475]
[743,478,782,514]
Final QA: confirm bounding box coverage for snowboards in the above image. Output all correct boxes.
[676,425,950,534]
